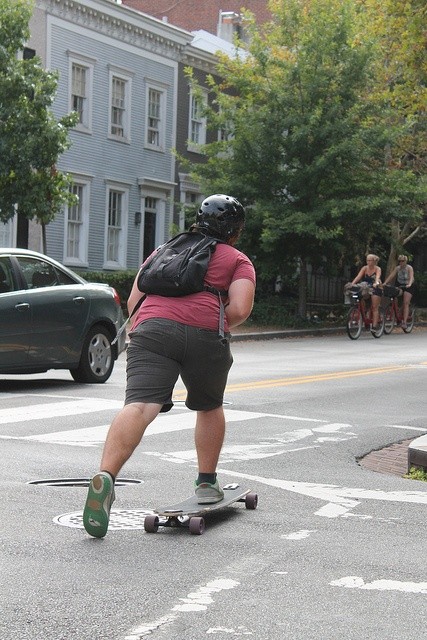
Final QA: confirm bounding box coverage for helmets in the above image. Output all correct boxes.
[197,193,245,242]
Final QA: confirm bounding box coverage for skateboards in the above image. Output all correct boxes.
[144,482,258,535]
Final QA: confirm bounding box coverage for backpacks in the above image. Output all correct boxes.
[110,230,228,346]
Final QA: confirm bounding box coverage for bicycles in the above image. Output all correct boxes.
[383,285,415,334]
[345,284,385,340]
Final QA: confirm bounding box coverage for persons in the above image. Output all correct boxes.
[350,254,382,333]
[83,194,256,539]
[385,255,413,329]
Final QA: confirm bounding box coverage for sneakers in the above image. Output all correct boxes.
[83,471,115,538]
[193,479,224,503]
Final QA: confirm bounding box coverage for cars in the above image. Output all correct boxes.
[0,248,126,384]
